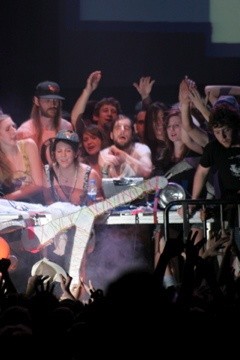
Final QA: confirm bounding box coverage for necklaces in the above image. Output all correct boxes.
[56,162,77,196]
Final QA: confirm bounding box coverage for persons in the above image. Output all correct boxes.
[177,101,240,260]
[0,231,240,360]
[0,71,240,209]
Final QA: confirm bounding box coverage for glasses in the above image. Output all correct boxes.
[164,124,180,129]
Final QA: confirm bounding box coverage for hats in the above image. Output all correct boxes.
[214,95,236,106]
[35,81,65,100]
[56,130,80,143]
